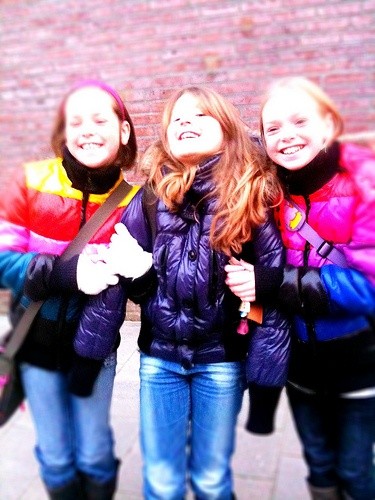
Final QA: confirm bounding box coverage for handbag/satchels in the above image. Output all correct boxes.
[0,330,25,427]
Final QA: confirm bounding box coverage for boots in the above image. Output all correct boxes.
[85,459,120,500]
[49,475,86,500]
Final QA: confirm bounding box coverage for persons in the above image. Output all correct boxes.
[70,86,291,500]
[226,76,375,500]
[0,81,153,500]
